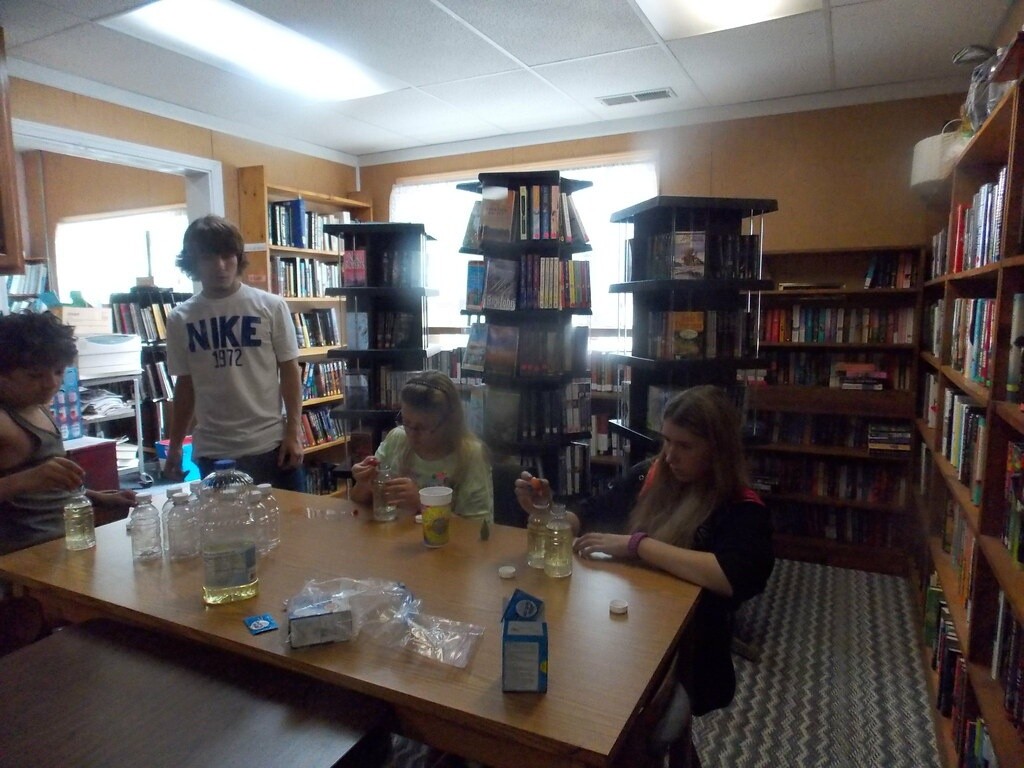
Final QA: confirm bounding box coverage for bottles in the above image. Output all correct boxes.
[199,459,259,604]
[168,493,200,562]
[64,484,96,551]
[130,493,162,561]
[527,497,552,569]
[252,491,270,561]
[161,487,183,551]
[545,505,573,578]
[373,465,397,521]
[257,483,281,550]
[188,482,201,555]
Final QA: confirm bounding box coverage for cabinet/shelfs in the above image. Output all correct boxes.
[0,20,1024,768]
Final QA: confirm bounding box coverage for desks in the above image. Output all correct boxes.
[0,614,398,768]
[1,485,703,768]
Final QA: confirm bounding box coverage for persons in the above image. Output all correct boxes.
[514,385,778,767]
[0,304,136,557]
[349,368,494,522]
[163,214,308,495]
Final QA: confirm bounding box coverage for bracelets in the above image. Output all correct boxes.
[627,532,648,562]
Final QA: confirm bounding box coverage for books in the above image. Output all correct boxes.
[913,166,1024,768]
[750,251,915,548]
[60,330,140,491]
[268,196,361,492]
[111,297,195,401]
[583,231,760,491]
[449,184,593,505]
[340,241,421,463]
[0,261,50,313]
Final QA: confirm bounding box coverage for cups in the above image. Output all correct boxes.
[419,487,453,548]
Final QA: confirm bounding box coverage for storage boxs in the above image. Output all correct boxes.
[50,306,112,335]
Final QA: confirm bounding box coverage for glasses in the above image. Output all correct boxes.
[393,410,447,435]
